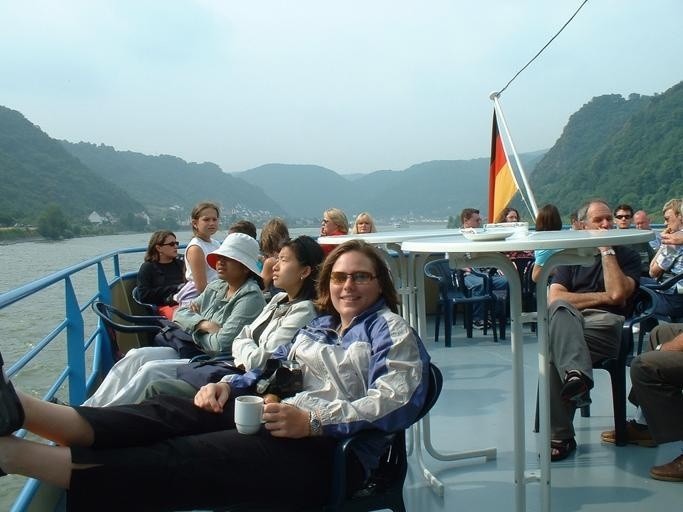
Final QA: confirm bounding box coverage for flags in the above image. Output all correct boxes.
[486,107,518,224]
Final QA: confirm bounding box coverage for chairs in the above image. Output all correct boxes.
[332,365,443,512]
[131,284,163,316]
[92,302,167,361]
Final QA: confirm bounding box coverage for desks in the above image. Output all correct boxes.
[401,227,657,512]
[316,223,497,499]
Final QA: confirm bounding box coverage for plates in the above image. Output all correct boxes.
[462,227,516,243]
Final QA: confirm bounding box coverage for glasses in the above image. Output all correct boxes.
[616,213,631,221]
[157,241,180,248]
[328,270,377,285]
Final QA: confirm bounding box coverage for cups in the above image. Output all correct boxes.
[233,394,269,437]
[509,221,529,244]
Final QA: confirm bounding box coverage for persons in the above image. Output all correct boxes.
[533,198,683,483]
[445,207,508,329]
[1,201,431,512]
[494,207,534,276]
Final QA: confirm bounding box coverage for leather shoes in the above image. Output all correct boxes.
[601,419,657,449]
[647,455,683,480]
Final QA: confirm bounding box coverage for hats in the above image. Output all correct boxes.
[205,232,262,286]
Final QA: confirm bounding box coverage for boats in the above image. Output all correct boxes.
[394,220,411,230]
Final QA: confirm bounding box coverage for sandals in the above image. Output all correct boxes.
[561,372,593,409]
[536,436,577,461]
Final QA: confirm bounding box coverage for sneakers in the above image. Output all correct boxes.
[0,351,25,435]
